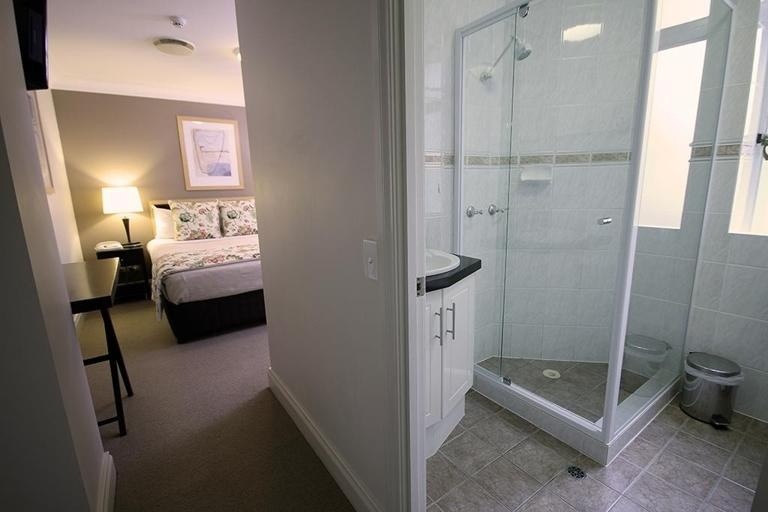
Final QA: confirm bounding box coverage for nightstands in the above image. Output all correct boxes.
[96,246,151,306]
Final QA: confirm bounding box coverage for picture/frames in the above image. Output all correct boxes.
[176,116,245,191]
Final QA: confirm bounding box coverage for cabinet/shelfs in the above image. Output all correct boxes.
[419,272,474,459]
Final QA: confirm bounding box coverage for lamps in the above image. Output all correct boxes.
[101,186,144,247]
[152,16,196,57]
[232,46,241,63]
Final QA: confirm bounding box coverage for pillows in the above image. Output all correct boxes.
[152,204,171,240]
[167,200,224,240]
[219,198,258,237]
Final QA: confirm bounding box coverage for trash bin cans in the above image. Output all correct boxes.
[679,351,743,426]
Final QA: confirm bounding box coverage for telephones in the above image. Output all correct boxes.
[94,241,125,252]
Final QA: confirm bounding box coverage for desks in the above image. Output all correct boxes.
[61,257,134,437]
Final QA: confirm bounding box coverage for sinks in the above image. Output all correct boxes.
[416,247,461,280]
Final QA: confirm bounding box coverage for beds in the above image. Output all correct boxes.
[147,197,267,344]
